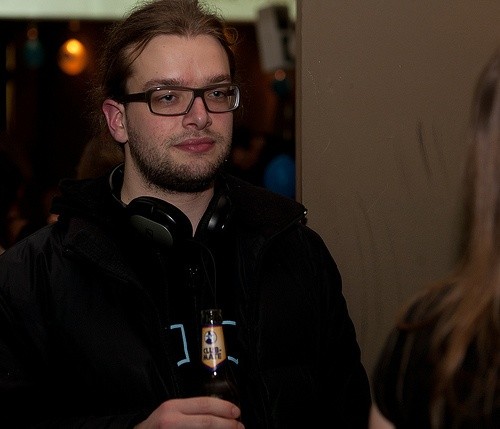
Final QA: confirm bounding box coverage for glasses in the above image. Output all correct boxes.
[113,83,241,117]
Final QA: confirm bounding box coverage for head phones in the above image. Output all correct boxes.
[109,161,234,276]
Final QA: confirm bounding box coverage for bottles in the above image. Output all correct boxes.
[199,308,237,403]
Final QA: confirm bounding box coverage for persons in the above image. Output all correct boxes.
[0,0,371,429]
[369,54,500,429]
